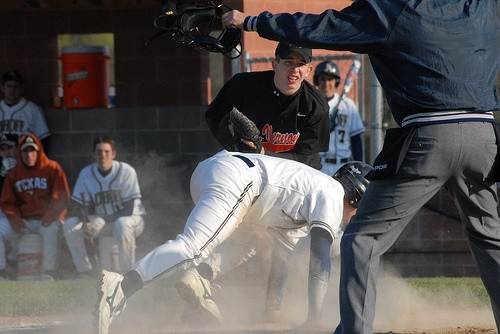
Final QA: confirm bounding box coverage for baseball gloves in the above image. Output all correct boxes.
[230,106,262,153]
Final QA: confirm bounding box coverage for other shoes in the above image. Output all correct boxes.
[0,275,9,280]
[77,272,91,280]
[44,271,59,281]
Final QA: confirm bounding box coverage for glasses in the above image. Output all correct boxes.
[0,146,13,150]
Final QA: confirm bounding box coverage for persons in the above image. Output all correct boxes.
[0,69,146,281]
[96,149,374,334]
[221,0,500,334]
[206,41,367,258]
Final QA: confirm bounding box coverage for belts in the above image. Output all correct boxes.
[232,155,260,207]
[319,157,349,164]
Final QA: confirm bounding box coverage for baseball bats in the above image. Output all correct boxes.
[77,191,105,282]
[328,59,362,123]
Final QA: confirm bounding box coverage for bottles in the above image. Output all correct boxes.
[111,245,119,271]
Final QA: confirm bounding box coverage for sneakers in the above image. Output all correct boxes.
[174,263,223,324]
[92,269,127,334]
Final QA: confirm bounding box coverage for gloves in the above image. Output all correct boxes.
[82,217,106,238]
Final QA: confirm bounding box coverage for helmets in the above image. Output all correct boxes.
[172,0,241,54]
[314,62,341,88]
[332,161,375,208]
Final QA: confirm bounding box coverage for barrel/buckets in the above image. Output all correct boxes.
[61,45,110,109]
[16,234,43,280]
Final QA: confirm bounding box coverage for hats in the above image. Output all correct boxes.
[275,42,312,63]
[19,136,39,151]
[0,134,18,147]
[2,70,23,84]
